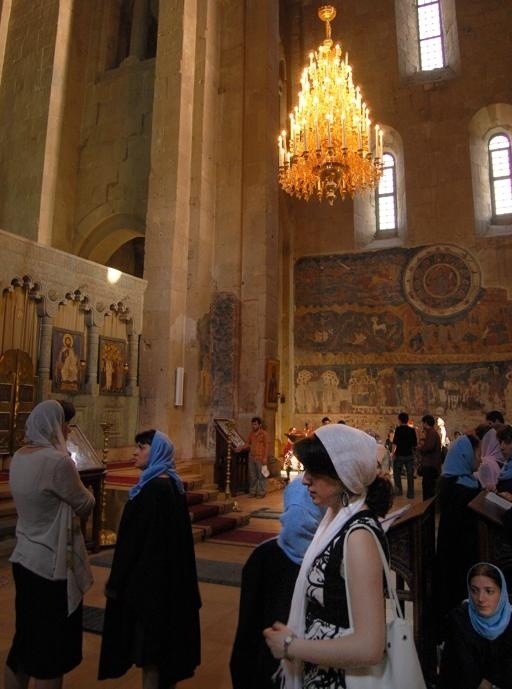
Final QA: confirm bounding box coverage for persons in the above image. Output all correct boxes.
[258,421,396,689]
[96,427,202,689]
[229,473,330,689]
[380,408,511,568]
[57,333,79,382]
[232,417,270,499]
[321,417,330,426]
[4,399,97,689]
[434,562,512,689]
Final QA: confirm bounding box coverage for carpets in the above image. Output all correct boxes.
[102,461,281,549]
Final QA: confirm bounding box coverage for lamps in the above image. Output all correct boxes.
[276,4,383,207]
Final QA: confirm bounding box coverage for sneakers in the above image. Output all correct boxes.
[407,492,416,499]
[393,489,404,497]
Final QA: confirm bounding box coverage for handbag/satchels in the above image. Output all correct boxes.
[335,619,427,688]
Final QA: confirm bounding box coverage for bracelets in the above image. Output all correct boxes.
[283,634,298,661]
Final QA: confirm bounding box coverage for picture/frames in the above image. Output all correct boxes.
[50,326,127,395]
